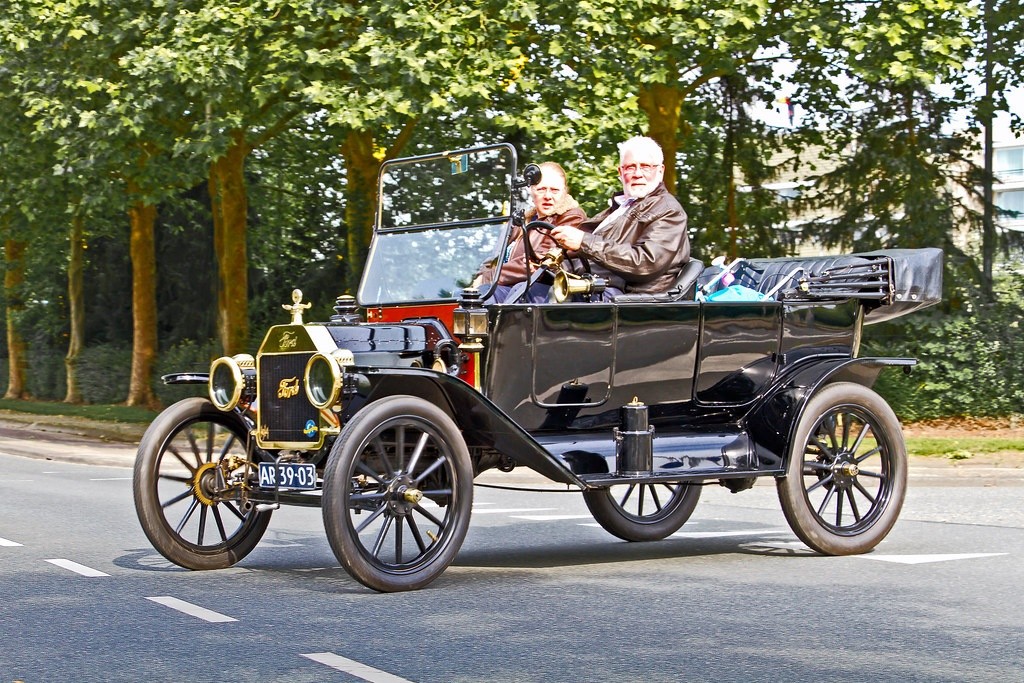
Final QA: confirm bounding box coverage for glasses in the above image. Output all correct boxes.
[621,165,659,174]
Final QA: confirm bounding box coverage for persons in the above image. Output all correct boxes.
[411,161,587,305]
[504,136,690,303]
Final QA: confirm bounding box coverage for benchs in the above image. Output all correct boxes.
[734,257,874,301]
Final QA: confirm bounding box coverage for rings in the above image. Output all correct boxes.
[561,241,564,245]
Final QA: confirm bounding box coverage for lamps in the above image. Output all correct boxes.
[330,295,363,323]
[453,288,489,353]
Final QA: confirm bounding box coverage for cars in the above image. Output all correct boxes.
[131,142,944,594]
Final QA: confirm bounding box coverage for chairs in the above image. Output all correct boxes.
[610,257,706,304]
